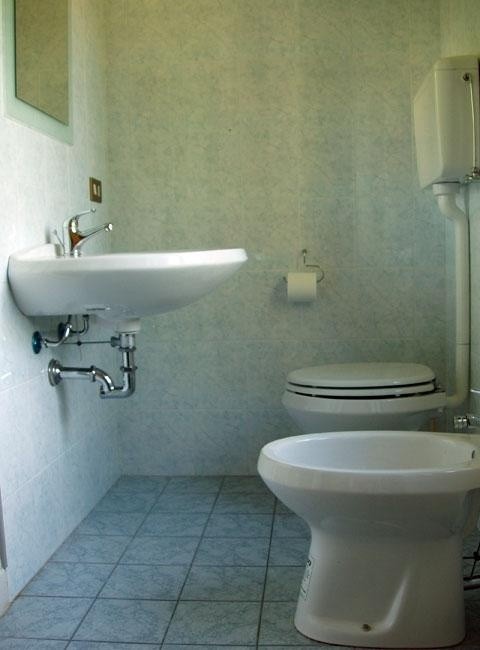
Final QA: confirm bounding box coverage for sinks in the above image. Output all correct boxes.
[3,247,249,316]
[257,431,479,543]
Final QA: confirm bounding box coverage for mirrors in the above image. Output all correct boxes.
[0,0,74,148]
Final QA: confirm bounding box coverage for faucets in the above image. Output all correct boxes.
[452,385,479,430]
[60,207,115,256]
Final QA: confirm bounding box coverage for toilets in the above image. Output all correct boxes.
[280,358,448,431]
